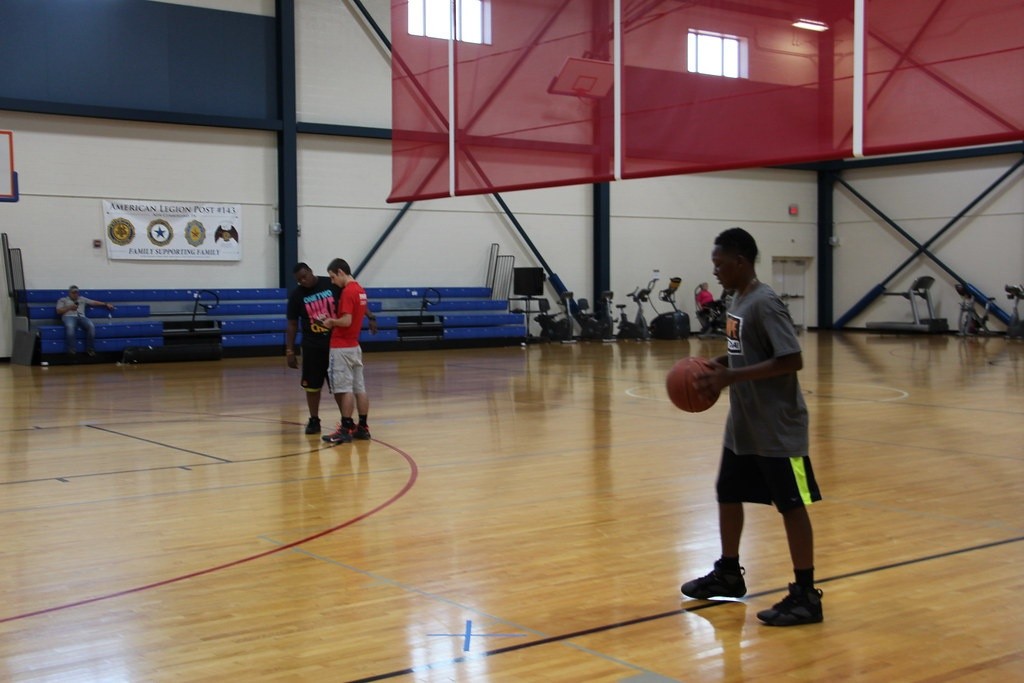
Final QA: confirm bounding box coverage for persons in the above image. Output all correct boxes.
[57,284,114,356]
[696,281,725,315]
[315,257,371,443]
[679,227,824,625]
[284,262,378,436]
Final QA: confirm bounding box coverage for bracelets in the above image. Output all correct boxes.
[286,348,295,355]
[105,302,107,306]
[369,316,376,320]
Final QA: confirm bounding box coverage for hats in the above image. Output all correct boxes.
[70,286,79,292]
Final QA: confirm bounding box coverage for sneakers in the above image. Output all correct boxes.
[681,560,746,599]
[322,423,353,443]
[352,423,370,440]
[757,583,823,626]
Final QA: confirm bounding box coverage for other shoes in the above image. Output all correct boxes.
[68,349,75,354]
[88,351,96,356]
[305,416,321,434]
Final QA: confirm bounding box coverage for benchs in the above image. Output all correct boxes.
[11,287,526,365]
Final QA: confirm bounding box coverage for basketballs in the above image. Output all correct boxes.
[667,355,721,413]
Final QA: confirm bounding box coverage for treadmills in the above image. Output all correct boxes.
[865,275,950,334]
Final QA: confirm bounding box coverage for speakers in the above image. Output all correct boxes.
[514,267,544,296]
